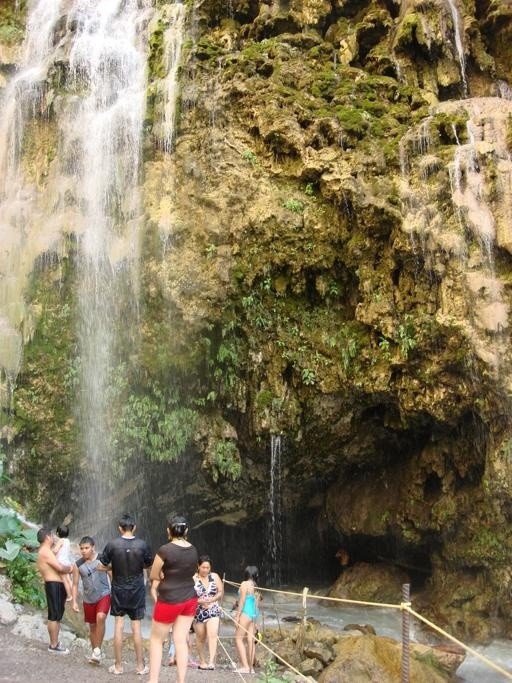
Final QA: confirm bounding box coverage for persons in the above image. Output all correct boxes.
[193,554,225,670]
[233,564,263,674]
[70,534,114,664]
[150,568,200,669]
[36,526,74,654]
[149,515,202,682]
[97,512,152,676]
[50,522,74,602]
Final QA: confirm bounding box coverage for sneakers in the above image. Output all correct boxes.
[90,647,150,676]
[198,664,215,669]
[233,668,255,674]
[48,645,69,655]
